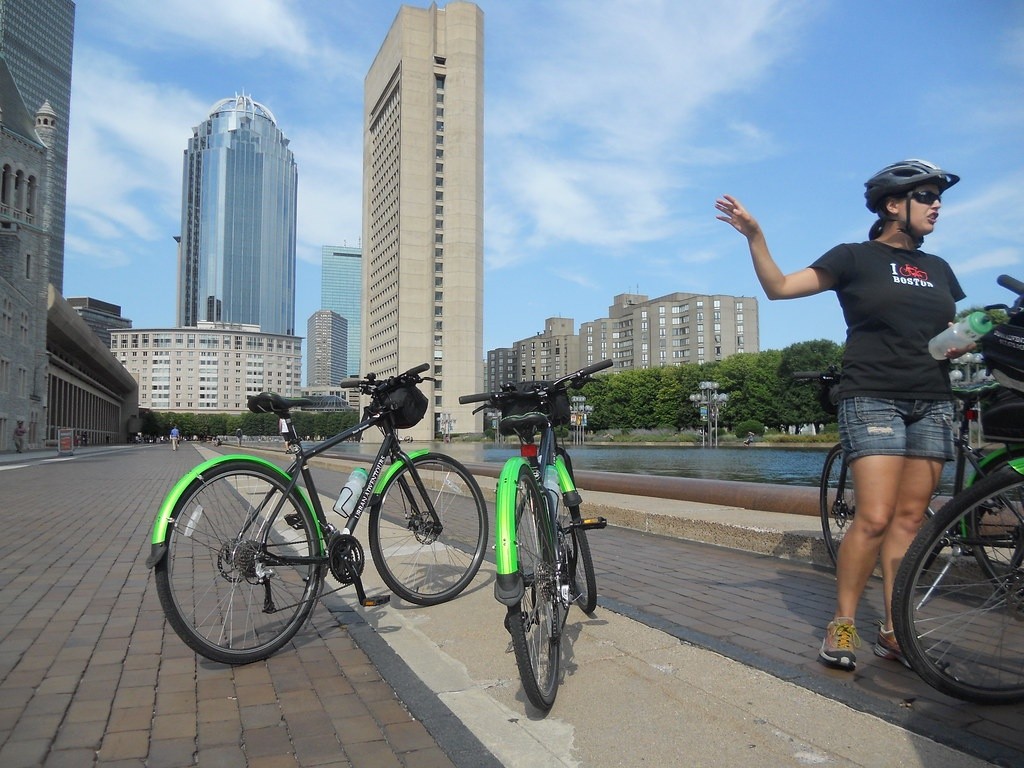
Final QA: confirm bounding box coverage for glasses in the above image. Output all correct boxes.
[910,191,941,205]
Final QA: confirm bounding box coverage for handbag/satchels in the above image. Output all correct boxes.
[498,380,571,434]
[366,382,429,428]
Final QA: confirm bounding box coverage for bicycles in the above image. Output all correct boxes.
[892,398,1024,704]
[145,361,490,666]
[458,358,614,711]
[793,365,1024,611]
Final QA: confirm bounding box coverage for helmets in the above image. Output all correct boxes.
[863,159,960,213]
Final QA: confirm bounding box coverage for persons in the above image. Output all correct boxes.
[716,157,974,669]
[13,421,25,453]
[236,427,242,446]
[171,426,179,451]
[278,418,292,454]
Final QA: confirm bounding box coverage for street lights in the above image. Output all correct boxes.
[569,396,594,446]
[689,381,727,447]
[436,408,456,444]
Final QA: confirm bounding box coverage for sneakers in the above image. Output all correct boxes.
[873,619,926,668]
[818,616,862,668]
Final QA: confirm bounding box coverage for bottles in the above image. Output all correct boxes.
[334,468,368,518]
[928,311,993,361]
[544,464,560,494]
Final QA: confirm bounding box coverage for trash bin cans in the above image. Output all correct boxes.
[57,428,73,454]
